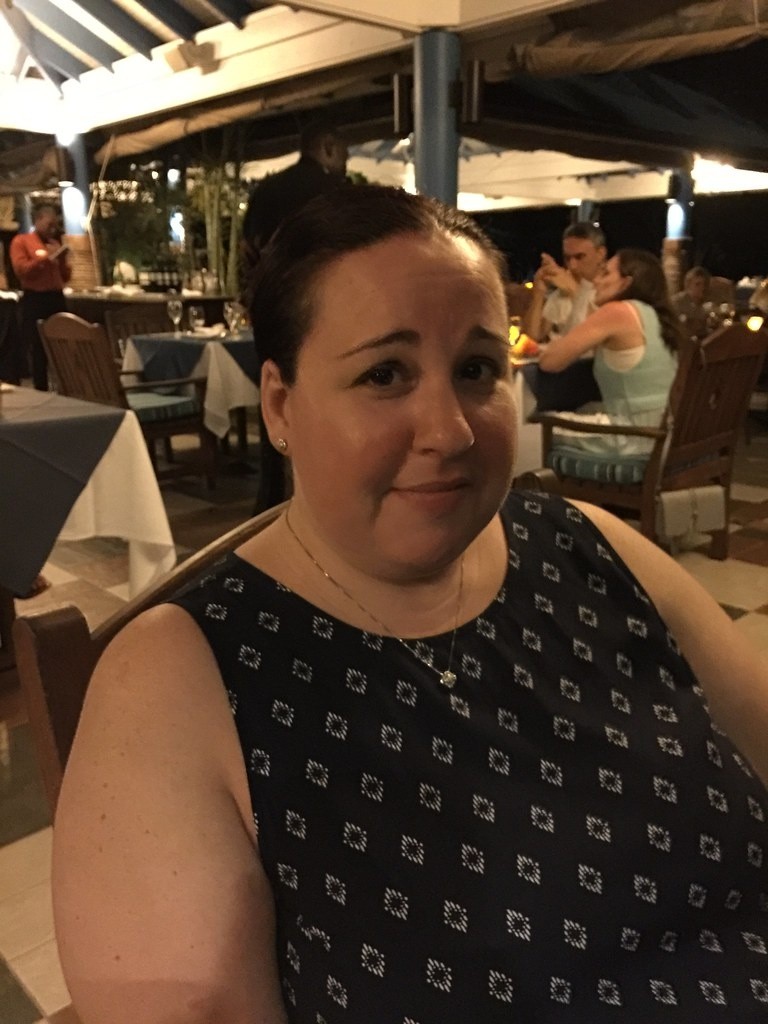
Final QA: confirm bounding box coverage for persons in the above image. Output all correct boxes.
[523,221,704,485]
[242,122,354,515]
[49,185,768,1024]
[10,204,71,391]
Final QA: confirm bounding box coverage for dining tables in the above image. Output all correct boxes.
[0,286,257,668]
[510,353,593,478]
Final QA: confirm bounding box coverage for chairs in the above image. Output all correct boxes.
[34,312,217,501]
[182,297,236,327]
[10,493,295,823]
[107,309,176,360]
[524,315,768,561]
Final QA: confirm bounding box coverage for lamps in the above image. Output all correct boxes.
[664,175,680,205]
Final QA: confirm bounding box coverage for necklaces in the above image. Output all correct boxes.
[284,498,467,690]
